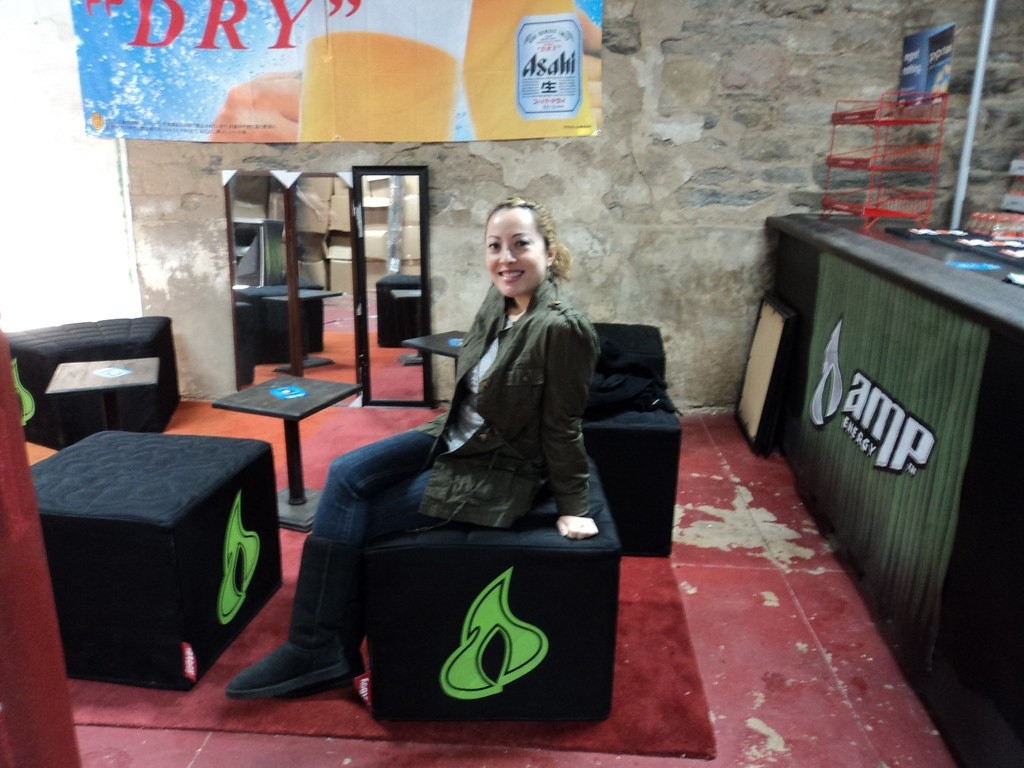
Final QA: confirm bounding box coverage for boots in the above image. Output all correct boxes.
[225,536,370,700]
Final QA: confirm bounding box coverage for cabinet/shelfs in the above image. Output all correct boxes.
[817,89,949,229]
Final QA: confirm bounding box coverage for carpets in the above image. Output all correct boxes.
[18,396,718,765]
[245,334,424,402]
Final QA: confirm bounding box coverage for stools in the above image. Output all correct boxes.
[234,278,324,384]
[576,324,684,562]
[27,429,283,696]
[376,274,423,347]
[366,447,622,725]
[5,316,183,451]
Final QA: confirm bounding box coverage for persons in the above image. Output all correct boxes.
[223,197,604,698]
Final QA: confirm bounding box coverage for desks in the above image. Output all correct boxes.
[212,374,363,533]
[264,296,293,375]
[46,357,162,431]
[402,330,467,382]
[303,290,341,369]
[763,212,1024,726]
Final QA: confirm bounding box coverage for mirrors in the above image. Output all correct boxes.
[352,164,433,407]
[222,168,302,394]
[287,170,363,394]
